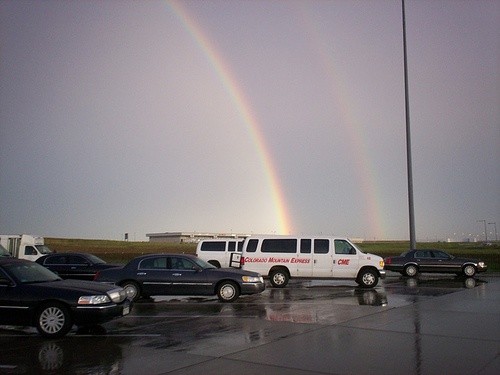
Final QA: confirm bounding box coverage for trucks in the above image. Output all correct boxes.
[0,234,53,262]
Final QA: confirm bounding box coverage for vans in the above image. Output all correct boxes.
[240,235,385,287]
[195,238,245,269]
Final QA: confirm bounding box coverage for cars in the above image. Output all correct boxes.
[34,252,123,280]
[95,254,264,303]
[0,256,132,339]
[384,249,488,276]
